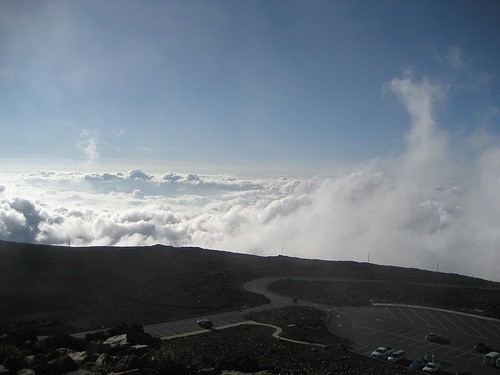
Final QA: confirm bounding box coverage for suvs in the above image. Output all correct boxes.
[197,319,215,329]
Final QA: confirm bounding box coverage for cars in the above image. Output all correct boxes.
[482,352,500,367]
[474,341,490,352]
[424,333,444,342]
[372,344,442,374]
